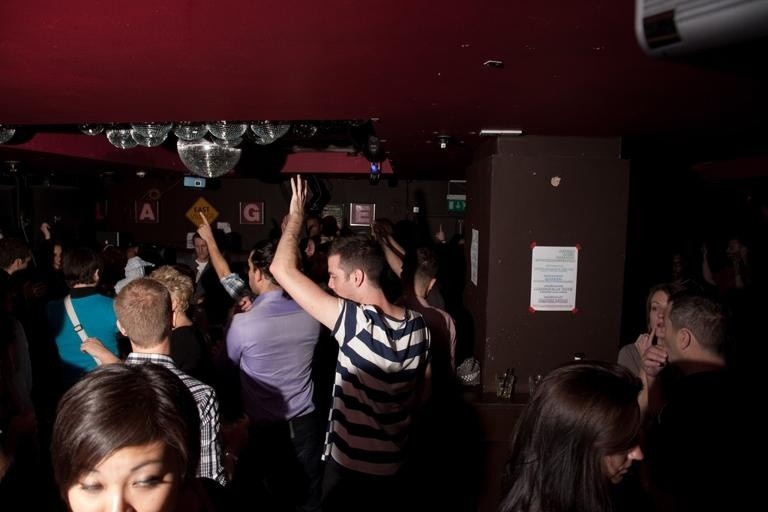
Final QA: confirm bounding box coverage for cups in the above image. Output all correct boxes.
[495,373,515,399]
[528,376,542,402]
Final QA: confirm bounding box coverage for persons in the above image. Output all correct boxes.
[652,289,768,512]
[498,360,646,512]
[2,174,459,511]
[617,283,688,421]
